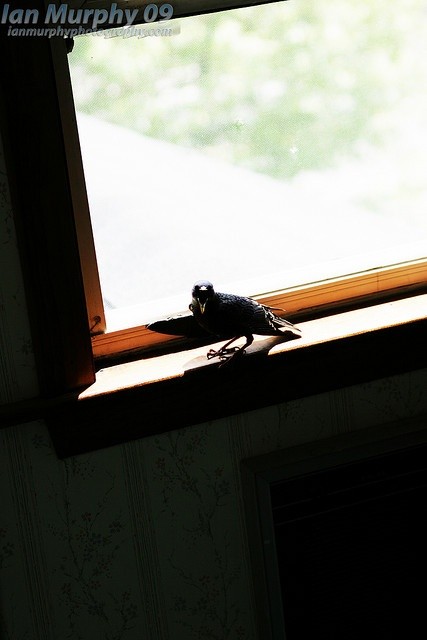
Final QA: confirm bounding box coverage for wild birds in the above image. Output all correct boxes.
[145,280,302,368]
